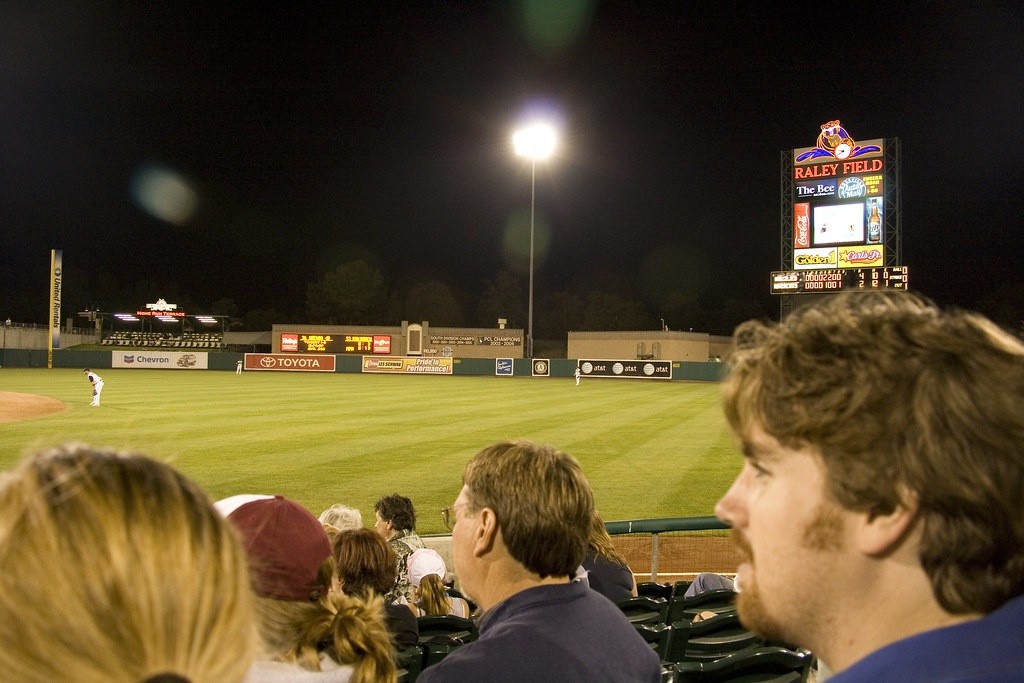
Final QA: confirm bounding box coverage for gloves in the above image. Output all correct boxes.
[93,390,96,396]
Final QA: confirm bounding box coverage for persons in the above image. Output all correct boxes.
[317,494,468,649]
[583,513,639,601]
[411,442,663,683]
[575,367,580,385]
[685,571,739,598]
[235,359,243,374]
[715,292,1024,683]
[211,494,395,683]
[0,446,254,683]
[83,369,104,406]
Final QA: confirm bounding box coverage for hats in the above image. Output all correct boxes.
[224,494,333,600]
[407,548,446,586]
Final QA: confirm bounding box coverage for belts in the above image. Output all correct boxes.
[100,379,103,381]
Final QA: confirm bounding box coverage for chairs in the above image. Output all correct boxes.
[102,330,223,350]
[385,575,817,683]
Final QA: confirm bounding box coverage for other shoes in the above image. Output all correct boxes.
[92,404,100,406]
[90,402,95,405]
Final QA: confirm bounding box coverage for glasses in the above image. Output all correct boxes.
[441,503,471,530]
